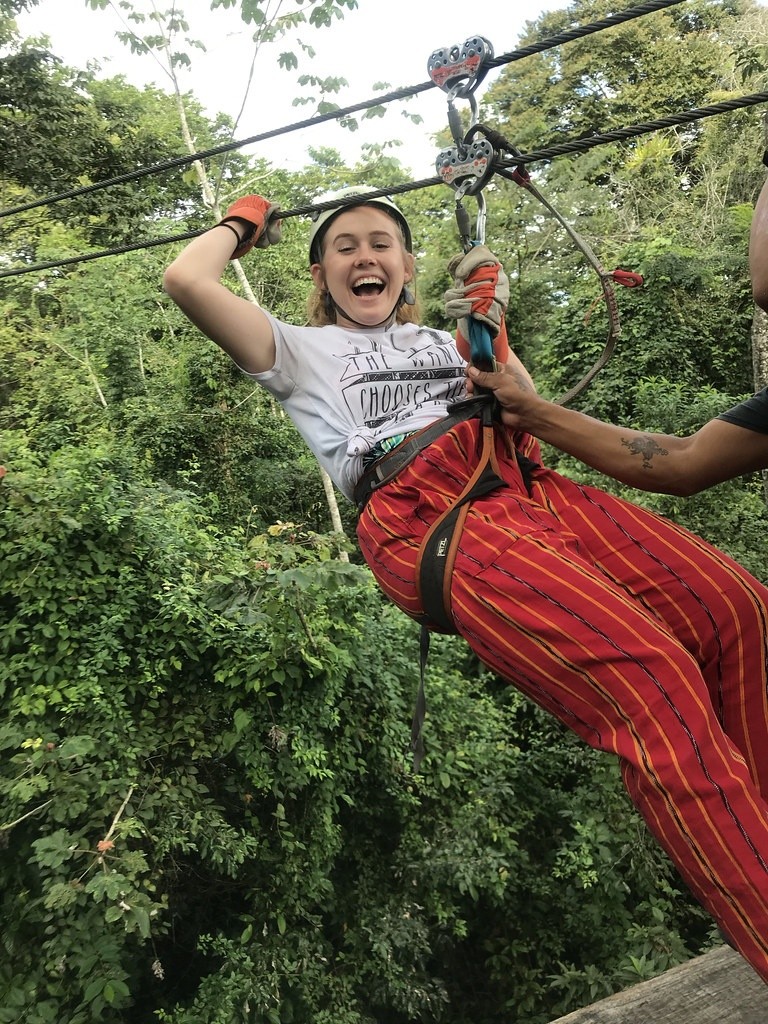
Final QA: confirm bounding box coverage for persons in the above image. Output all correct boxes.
[158,182,768,983]
[464,170,768,501]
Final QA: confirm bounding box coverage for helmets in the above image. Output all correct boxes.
[307,184,413,264]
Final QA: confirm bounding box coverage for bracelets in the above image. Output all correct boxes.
[213,223,241,251]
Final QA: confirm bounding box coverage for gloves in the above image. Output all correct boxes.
[215,193,283,260]
[443,244,510,369]
